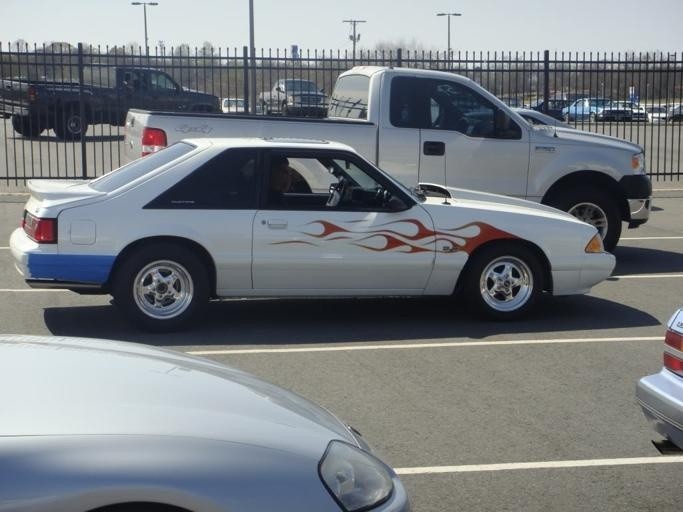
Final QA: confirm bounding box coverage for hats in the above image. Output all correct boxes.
[634,308,683,454]
[459,107,575,137]
[0,333,413,512]
[220,97,252,115]
[6,137,618,336]
[495,91,683,124]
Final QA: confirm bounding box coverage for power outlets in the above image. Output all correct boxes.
[259,77,331,119]
[124,65,653,255]
[0,65,225,141]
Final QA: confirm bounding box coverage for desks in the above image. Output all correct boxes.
[131,2,159,64]
[437,11,462,68]
